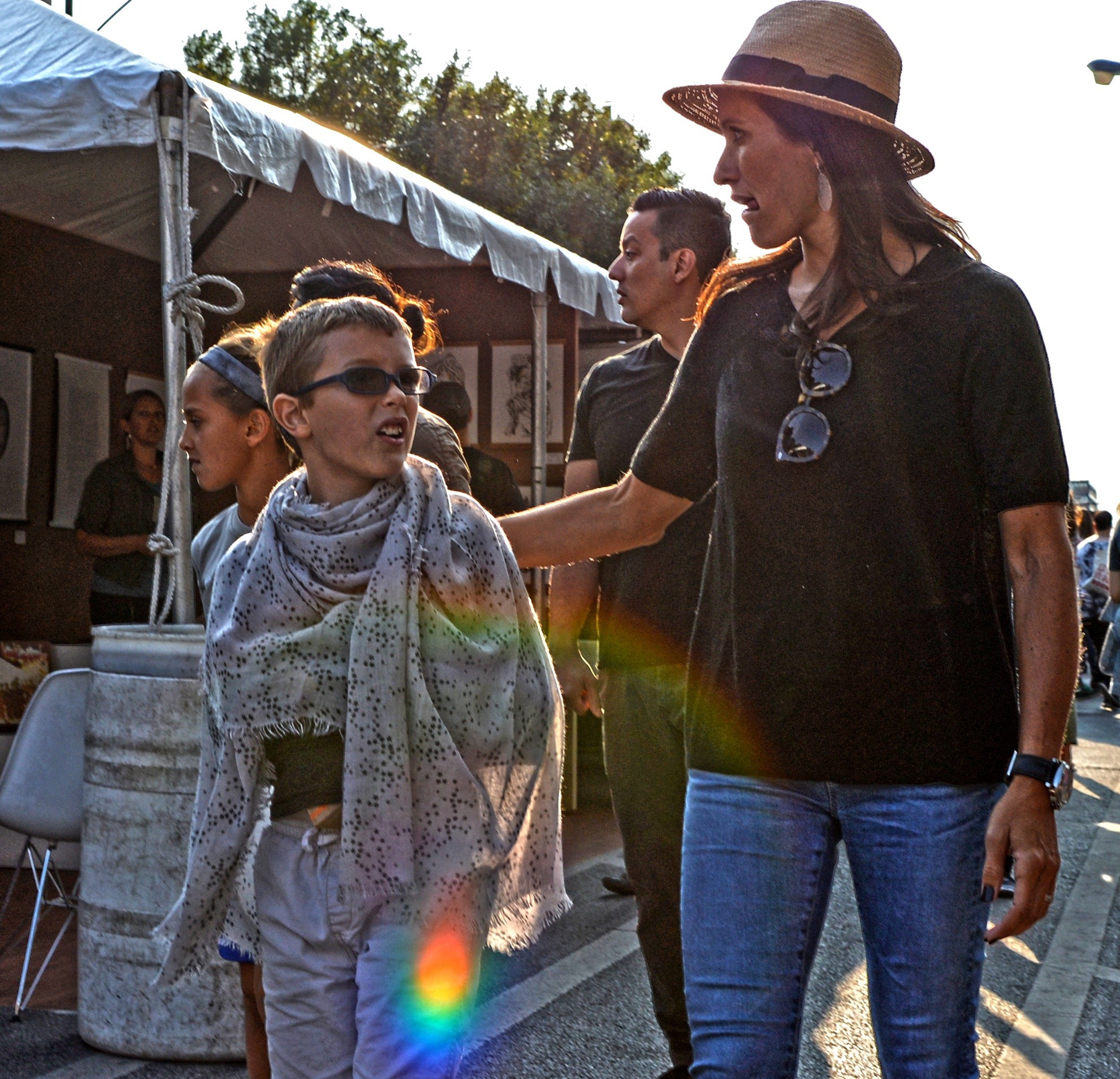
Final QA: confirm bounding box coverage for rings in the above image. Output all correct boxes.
[1043,894,1054,905]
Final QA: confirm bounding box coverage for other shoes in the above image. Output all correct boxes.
[998,875,1015,898]
[602,873,635,896]
[1102,693,1120,710]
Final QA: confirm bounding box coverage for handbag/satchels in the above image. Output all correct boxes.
[1080,549,1110,599]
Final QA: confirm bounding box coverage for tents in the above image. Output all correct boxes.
[0,0,652,812]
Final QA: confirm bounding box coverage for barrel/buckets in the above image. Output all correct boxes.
[76,623,247,1063]
[49,639,90,672]
[0,640,50,725]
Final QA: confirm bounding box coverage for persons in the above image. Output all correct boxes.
[79,192,1120,1079]
[477,0,1079,1079]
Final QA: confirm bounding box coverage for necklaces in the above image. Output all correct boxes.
[135,457,158,471]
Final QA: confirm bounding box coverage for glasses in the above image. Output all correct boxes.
[290,367,437,396]
[774,339,852,463]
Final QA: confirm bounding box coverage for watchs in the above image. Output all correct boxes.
[1000,751,1074,812]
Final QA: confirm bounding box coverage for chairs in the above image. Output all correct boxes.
[0,668,90,1022]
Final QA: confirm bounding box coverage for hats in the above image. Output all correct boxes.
[662,0,935,182]
[423,381,470,417]
